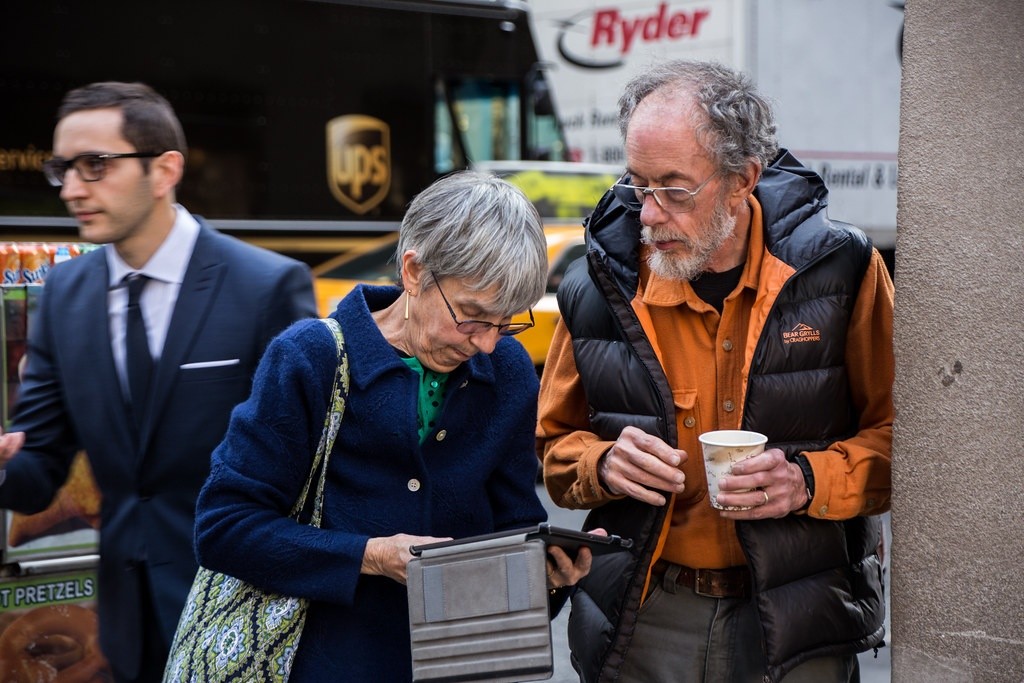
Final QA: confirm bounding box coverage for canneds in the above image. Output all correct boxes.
[0,241,104,285]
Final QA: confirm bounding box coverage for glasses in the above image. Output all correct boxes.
[609,161,730,213]
[430,267,535,337]
[42,149,164,187]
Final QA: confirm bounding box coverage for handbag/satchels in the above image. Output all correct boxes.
[162,317,349,683]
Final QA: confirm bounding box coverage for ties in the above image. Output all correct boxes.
[122,273,154,438]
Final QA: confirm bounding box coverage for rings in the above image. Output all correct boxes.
[763,490,769,506]
[548,570,555,578]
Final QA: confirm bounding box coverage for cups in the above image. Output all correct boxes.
[699,430,768,511]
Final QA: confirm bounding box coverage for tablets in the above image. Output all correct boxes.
[409,522,634,563]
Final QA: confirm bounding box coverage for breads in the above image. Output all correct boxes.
[0,602,114,683]
[7,449,102,547]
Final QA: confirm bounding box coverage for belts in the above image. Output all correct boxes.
[653,558,749,598]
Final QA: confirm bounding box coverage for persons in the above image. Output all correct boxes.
[0,83,319,683]
[193,170,607,683]
[537,61,895,683]
